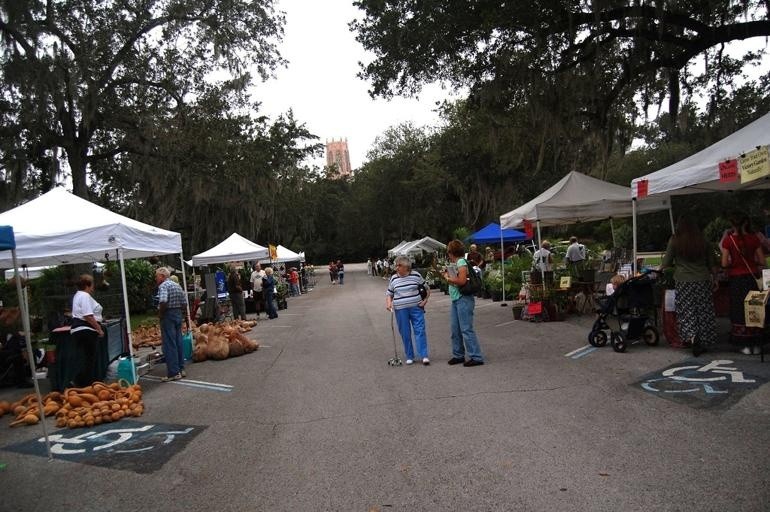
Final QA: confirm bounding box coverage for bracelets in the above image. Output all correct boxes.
[445,276,449,282]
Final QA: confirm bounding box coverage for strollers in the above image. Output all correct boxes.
[590,268,664,354]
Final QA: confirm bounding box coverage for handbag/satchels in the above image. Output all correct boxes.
[418,284,427,299]
[756,277,763,291]
[459,270,482,296]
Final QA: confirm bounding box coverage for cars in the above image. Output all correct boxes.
[523,239,603,264]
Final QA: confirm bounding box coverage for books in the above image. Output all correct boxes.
[447,266,457,278]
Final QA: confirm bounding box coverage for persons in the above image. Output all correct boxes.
[385,256,431,364]
[68,274,104,386]
[329,260,345,285]
[249,264,270,321]
[155,265,188,382]
[532,239,554,282]
[227,264,249,321]
[566,237,586,277]
[265,267,278,319]
[367,251,444,280]
[467,242,534,268]
[660,212,765,355]
[610,274,626,291]
[274,262,317,297]
[441,239,485,366]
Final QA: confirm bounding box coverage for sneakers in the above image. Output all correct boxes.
[161,369,186,382]
[406,358,482,367]
[740,346,761,355]
[691,334,701,357]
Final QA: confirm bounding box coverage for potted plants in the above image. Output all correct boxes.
[425,258,449,295]
[475,238,603,322]
[276,284,287,310]
